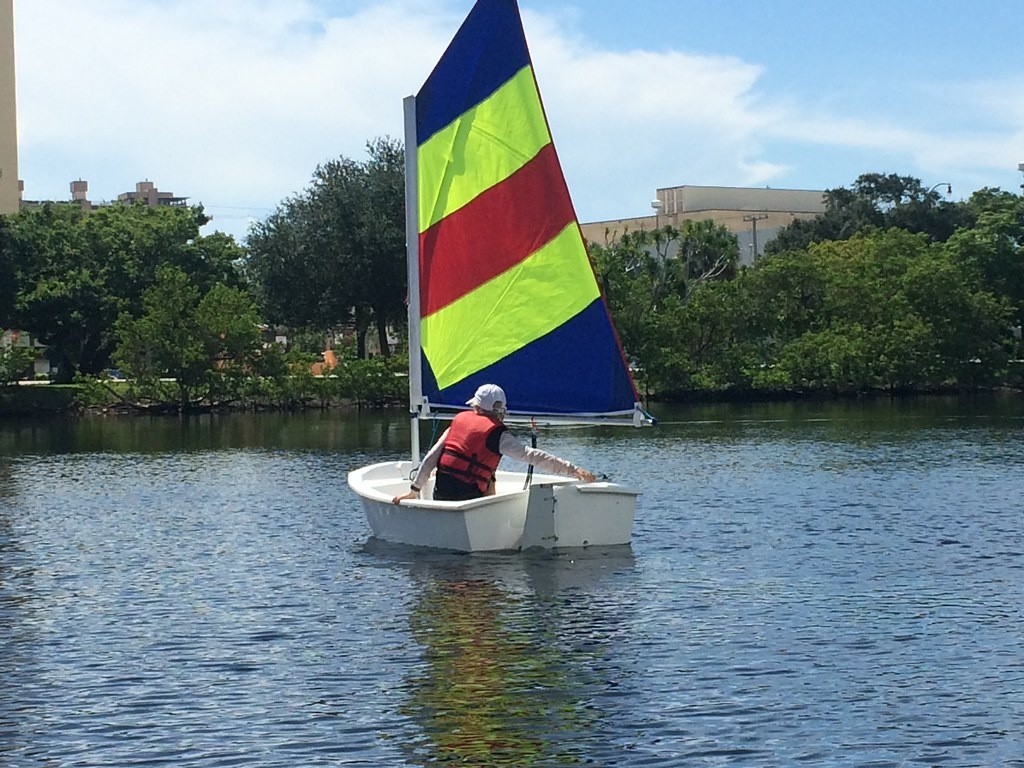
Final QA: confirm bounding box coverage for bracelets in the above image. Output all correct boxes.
[411,485,420,493]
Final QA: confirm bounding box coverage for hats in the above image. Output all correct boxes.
[466,384,507,413]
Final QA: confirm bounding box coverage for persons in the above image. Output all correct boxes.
[390,382,595,505]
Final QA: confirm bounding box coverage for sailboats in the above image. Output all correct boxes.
[346,0,652,553]
